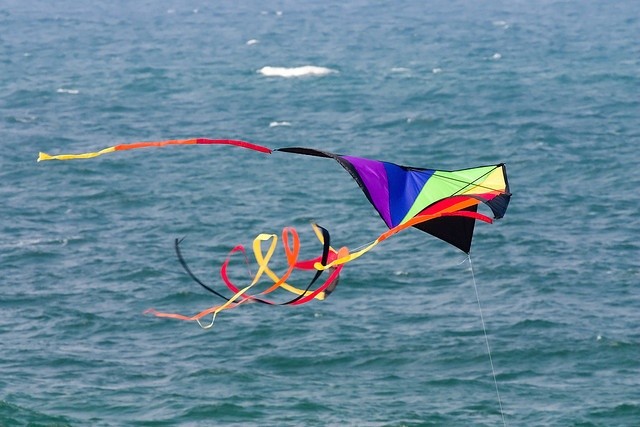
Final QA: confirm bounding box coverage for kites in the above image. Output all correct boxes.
[37,139,513,329]
